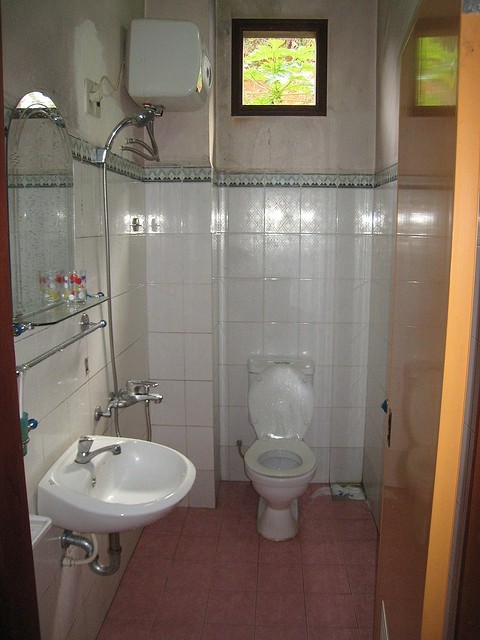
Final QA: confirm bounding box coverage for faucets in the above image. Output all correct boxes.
[75,435,121,464]
[117,379,163,409]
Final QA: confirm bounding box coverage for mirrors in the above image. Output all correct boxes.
[6,91,76,319]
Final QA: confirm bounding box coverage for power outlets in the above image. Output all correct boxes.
[81,77,95,116]
[94,82,102,119]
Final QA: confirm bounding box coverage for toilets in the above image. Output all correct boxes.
[244,356,318,542]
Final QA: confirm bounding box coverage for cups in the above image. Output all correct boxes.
[37,268,65,305]
[63,268,86,304]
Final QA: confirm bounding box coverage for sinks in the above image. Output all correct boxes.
[37,435,196,534]
